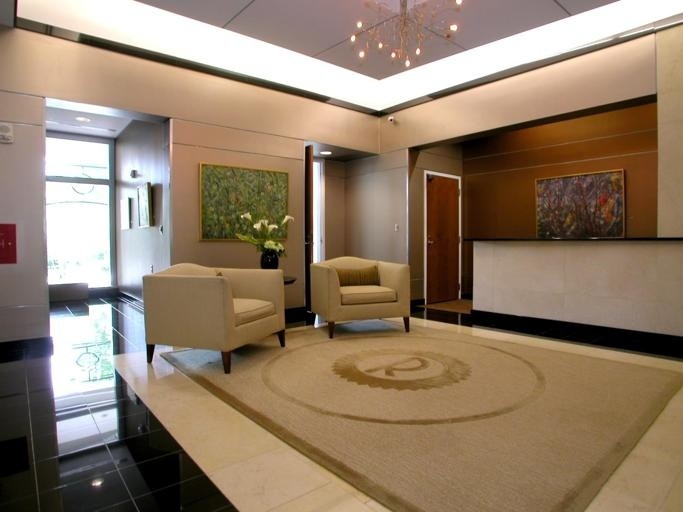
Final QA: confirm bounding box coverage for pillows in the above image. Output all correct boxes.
[335,266,381,285]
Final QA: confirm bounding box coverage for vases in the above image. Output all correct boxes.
[260,250,279,269]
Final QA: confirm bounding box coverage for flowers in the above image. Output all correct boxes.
[237,211,293,258]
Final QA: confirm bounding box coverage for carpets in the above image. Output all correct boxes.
[159,318,683,512]
[416,298,471,315]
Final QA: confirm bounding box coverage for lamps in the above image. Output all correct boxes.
[349,1,462,68]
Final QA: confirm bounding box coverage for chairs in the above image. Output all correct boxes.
[143,262,287,373]
[310,256,411,338]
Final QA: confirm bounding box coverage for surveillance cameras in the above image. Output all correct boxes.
[388,117,394,123]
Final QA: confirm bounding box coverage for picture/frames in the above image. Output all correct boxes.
[534,168,626,241]
[121,197,131,230]
[136,182,153,228]
[199,161,290,242]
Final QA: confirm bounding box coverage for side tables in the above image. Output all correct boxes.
[283,276,298,284]
